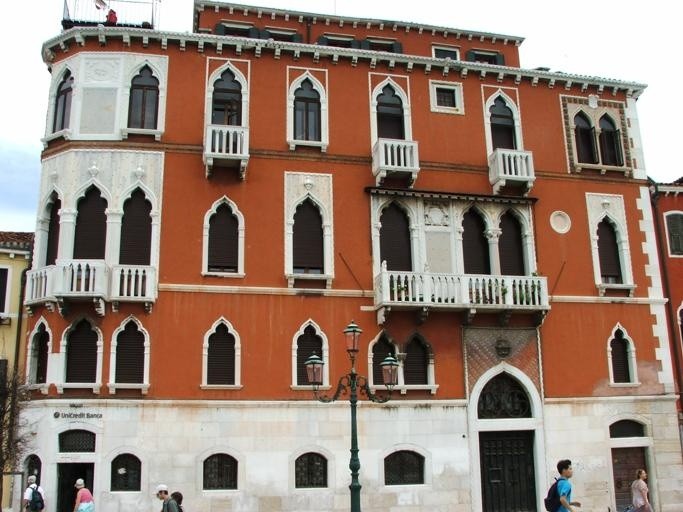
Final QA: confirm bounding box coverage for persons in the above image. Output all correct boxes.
[555,459,582,512]
[170,491,183,512]
[631,468,654,512]
[73,478,95,512]
[153,484,179,512]
[23,475,47,512]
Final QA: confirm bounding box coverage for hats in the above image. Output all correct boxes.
[75,478,85,488]
[152,484,168,495]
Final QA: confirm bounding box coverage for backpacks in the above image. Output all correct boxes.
[544,478,571,512]
[27,486,44,511]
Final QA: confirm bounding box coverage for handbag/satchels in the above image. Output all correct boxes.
[76,502,96,512]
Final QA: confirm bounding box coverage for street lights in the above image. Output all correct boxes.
[306,318,400,512]
